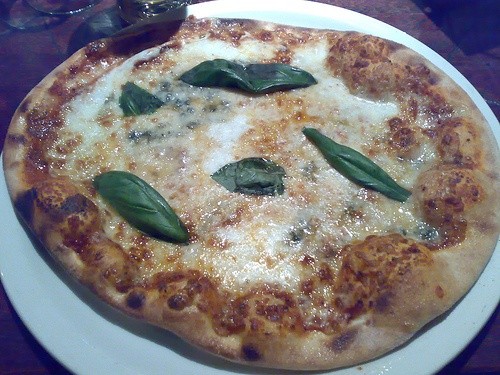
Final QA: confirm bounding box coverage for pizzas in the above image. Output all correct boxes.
[3,18,500,369]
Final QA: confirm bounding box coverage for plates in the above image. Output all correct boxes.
[1,0,499,375]
